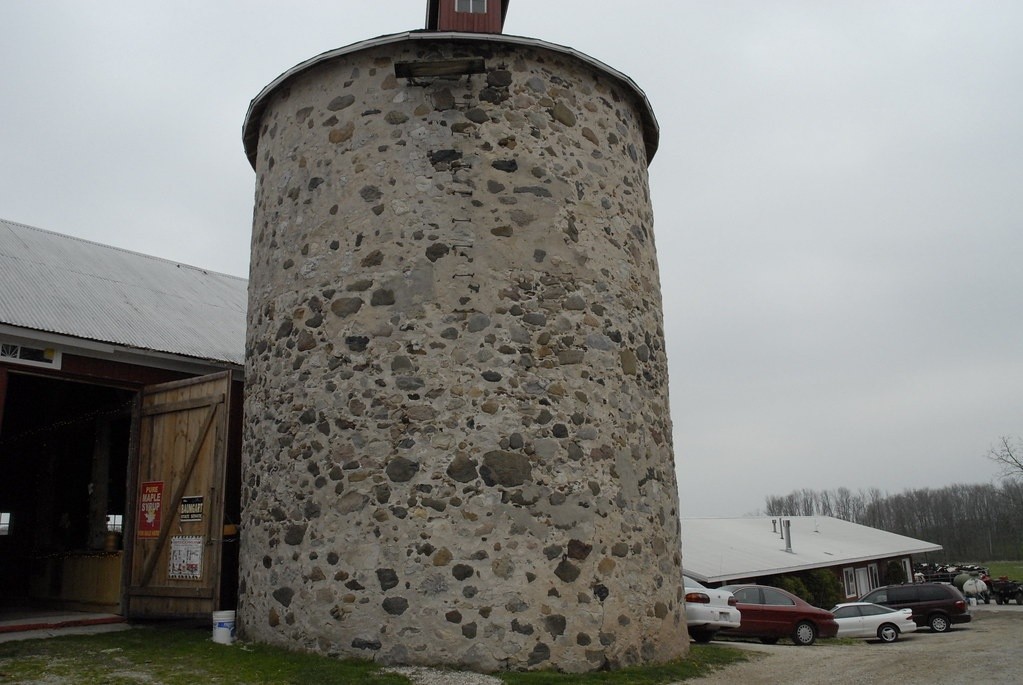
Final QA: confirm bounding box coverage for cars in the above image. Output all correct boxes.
[829,603,917,643]
[714,585,840,647]
[682,575,742,644]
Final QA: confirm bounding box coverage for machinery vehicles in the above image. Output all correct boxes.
[981,575,1023,606]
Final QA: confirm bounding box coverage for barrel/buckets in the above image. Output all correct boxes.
[969,597,977,606]
[211,610,235,644]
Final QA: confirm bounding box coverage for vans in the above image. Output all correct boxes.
[856,581,974,633]
